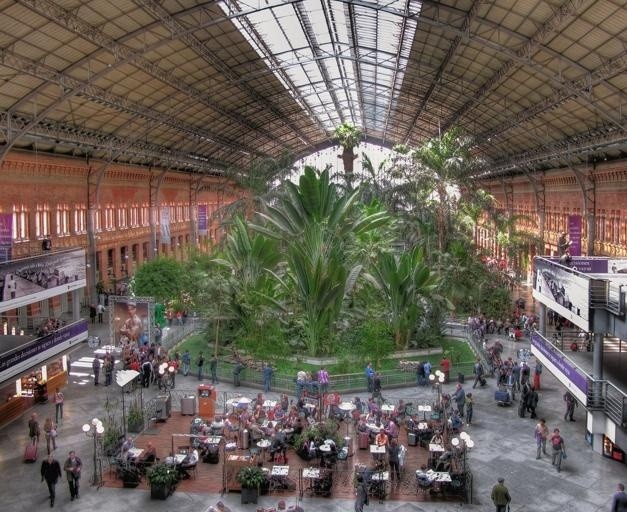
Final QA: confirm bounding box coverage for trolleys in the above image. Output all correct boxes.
[507,327,522,342]
[497,381,514,406]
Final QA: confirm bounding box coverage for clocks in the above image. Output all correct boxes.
[87,335,101,349]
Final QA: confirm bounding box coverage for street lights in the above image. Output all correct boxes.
[158,362,174,393]
[82,418,104,486]
[450,431,473,503]
[429,369,445,423]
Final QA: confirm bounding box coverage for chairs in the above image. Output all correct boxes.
[221,396,469,505]
[190,416,230,465]
[106,434,198,501]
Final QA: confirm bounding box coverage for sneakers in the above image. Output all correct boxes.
[69,493,81,501]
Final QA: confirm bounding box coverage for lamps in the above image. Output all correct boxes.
[452,432,473,500]
[82,418,104,487]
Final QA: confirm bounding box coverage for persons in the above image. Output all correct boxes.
[557,231,574,255]
[611,483,627,512]
[41,234,52,250]
[560,250,572,267]
[8,275,17,299]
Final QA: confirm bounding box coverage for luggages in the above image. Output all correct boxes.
[495,390,509,402]
[23,446,39,463]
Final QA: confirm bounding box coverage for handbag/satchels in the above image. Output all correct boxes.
[50,430,58,439]
[562,452,567,459]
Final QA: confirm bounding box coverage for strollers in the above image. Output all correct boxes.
[432,392,452,415]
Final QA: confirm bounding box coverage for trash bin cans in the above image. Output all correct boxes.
[407,432,416,446]
[344,436,353,457]
[241,430,250,450]
